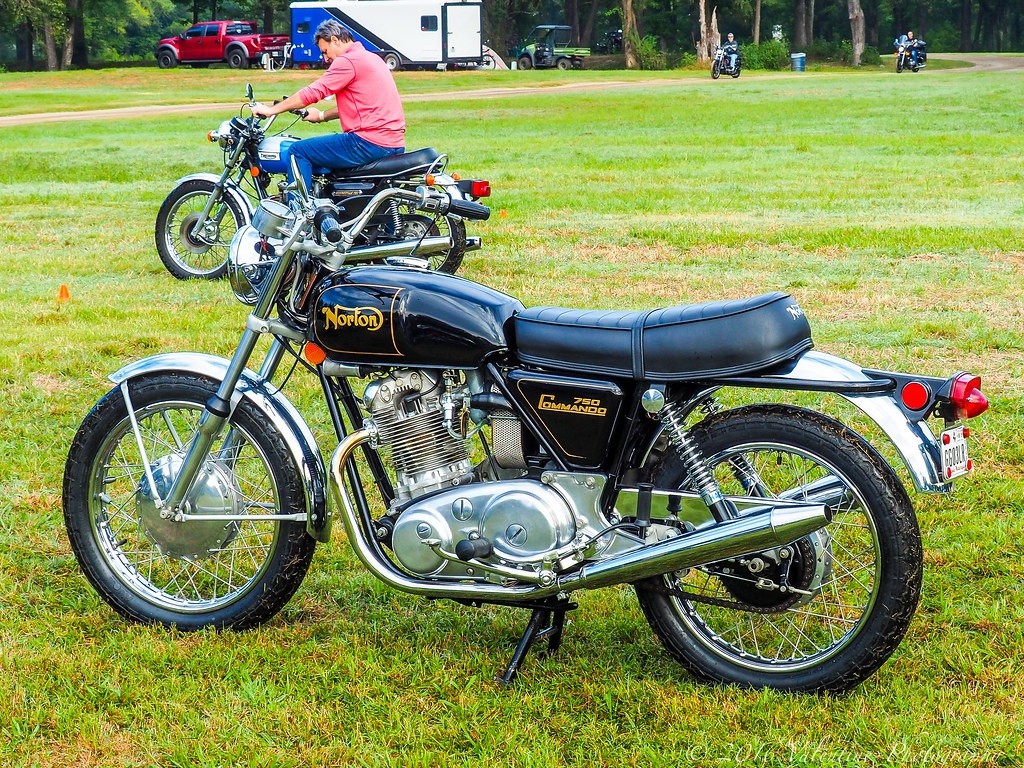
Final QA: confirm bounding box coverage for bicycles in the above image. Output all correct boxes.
[453,48,496,70]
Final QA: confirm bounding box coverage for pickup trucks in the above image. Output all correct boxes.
[154,20,293,70]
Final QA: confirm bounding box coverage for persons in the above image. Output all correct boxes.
[906,32,919,66]
[722,33,740,70]
[250,23,406,206]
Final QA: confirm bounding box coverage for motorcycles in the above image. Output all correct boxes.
[710,44,742,79]
[515,24,591,70]
[61,152,989,687]
[894,35,927,73]
[154,83,491,302]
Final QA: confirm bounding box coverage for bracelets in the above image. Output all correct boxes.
[319,111,327,122]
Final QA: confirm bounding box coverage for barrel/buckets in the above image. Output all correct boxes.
[792,53,806,71]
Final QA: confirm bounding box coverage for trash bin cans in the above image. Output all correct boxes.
[791,52,806,73]
